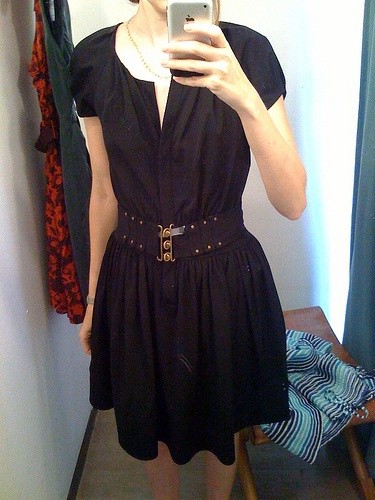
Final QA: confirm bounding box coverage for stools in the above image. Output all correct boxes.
[232,304,375,499]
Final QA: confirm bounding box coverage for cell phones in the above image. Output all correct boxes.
[167,0,213,77]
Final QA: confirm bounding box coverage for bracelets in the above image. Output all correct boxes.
[86,295,95,305]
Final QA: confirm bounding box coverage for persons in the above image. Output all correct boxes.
[64,0,308,500]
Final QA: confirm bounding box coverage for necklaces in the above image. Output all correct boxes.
[127,15,172,79]
